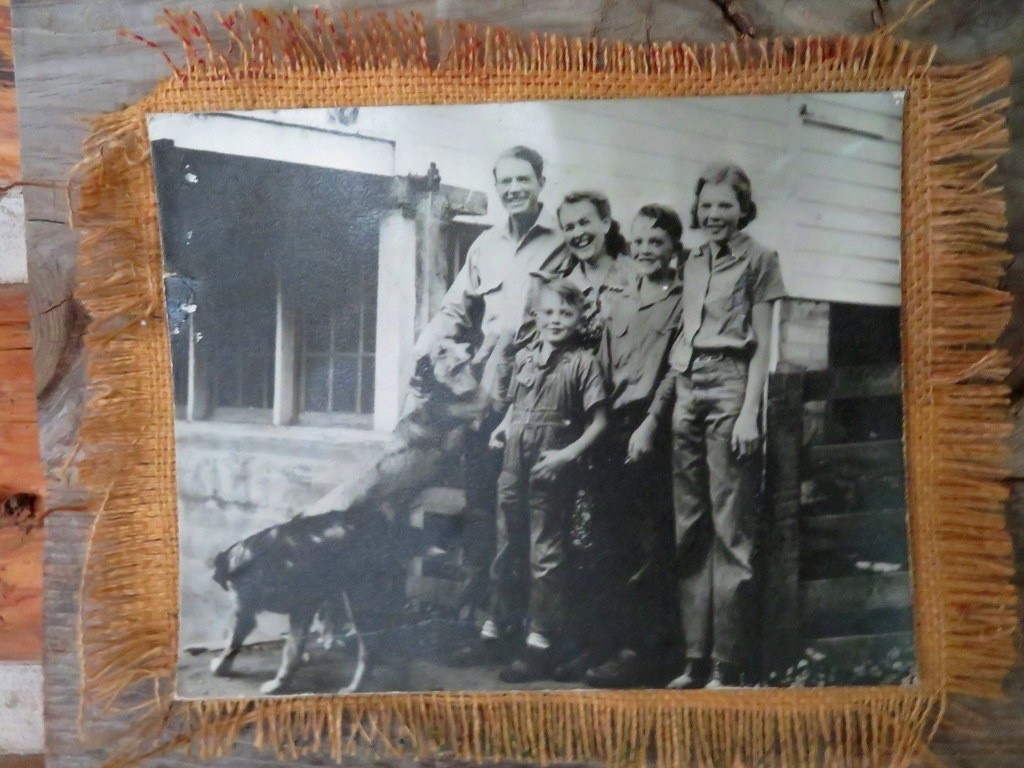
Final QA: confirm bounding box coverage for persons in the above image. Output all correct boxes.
[402,144,796,686]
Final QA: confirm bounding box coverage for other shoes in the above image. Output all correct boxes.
[664,661,748,688]
[449,634,499,665]
[551,648,667,686]
[498,644,556,683]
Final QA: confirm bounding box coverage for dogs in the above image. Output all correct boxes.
[210,507,392,694]
[295,323,504,648]
